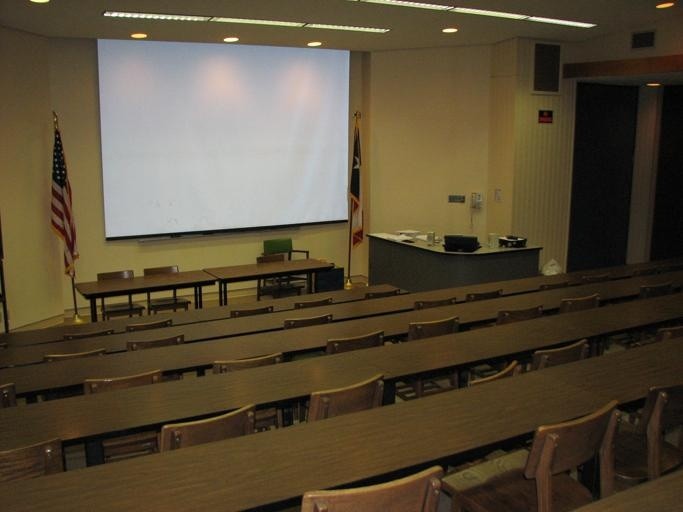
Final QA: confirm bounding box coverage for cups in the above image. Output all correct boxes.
[487,233,496,248]
[426,232,434,245]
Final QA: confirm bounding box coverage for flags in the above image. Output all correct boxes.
[348,119,364,247]
[48,125,80,278]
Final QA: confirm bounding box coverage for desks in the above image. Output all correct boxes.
[369,229,544,296]
[71,258,333,320]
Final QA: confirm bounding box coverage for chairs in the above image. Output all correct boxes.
[0,229,683,511]
